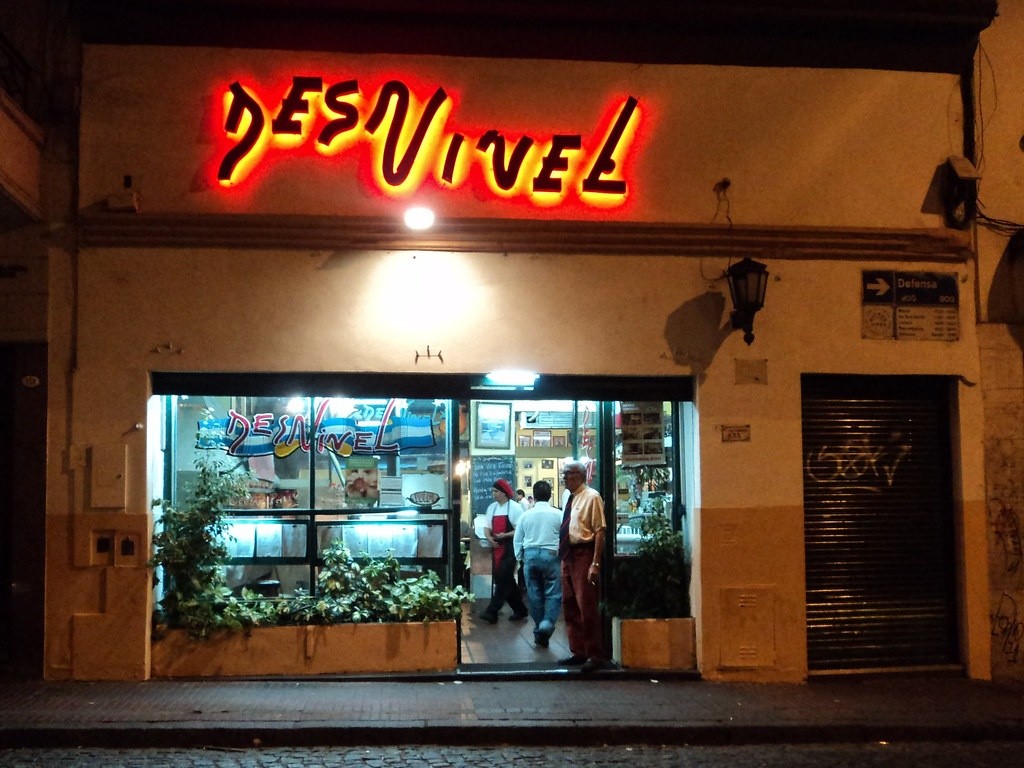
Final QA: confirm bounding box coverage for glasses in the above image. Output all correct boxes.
[560,471,580,477]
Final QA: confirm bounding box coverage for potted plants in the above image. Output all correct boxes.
[611,466,696,670]
[150,406,476,677]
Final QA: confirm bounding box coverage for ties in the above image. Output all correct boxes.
[559,493,575,562]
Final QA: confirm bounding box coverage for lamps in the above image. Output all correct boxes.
[724,254,767,347]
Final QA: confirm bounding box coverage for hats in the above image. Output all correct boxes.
[492,480,514,498]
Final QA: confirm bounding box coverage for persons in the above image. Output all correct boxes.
[557,460,609,674]
[516,489,530,511]
[346,469,378,499]
[513,478,564,647]
[478,480,530,623]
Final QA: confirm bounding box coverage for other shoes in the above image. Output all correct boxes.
[533,629,549,648]
[509,611,528,621]
[559,655,586,665]
[582,658,608,671]
[479,613,498,624]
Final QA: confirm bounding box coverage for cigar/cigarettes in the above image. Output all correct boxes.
[591,581,596,585]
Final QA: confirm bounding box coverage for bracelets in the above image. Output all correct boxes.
[591,562,601,568]
